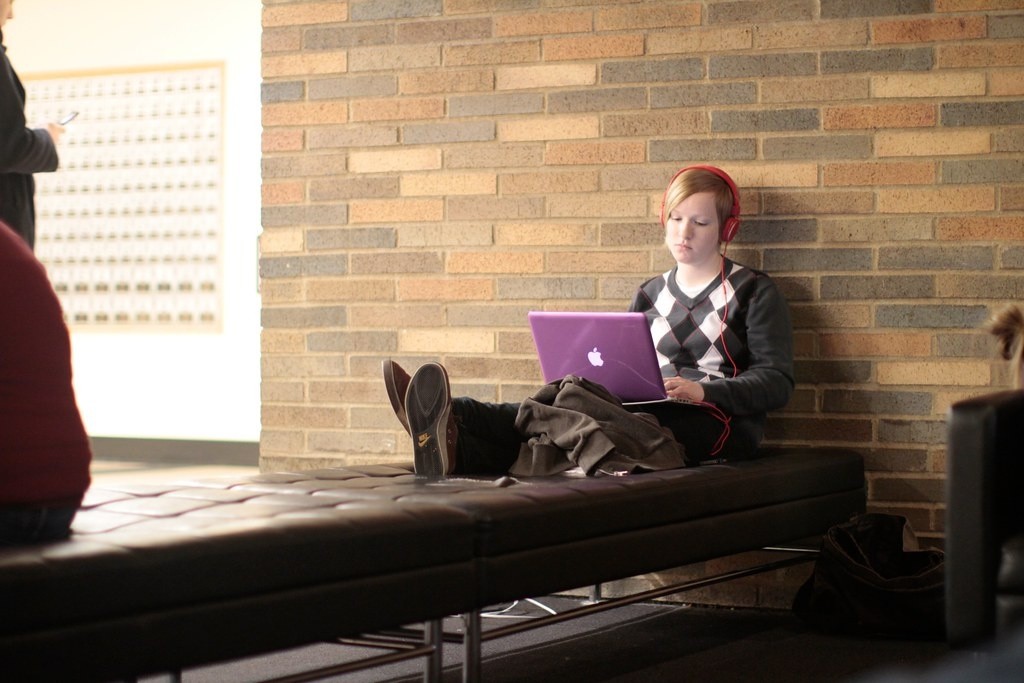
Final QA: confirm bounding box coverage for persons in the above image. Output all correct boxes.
[0,220,93,548]
[382,165,794,478]
[0,0,67,254]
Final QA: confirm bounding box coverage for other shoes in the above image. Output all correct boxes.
[384,360,450,483]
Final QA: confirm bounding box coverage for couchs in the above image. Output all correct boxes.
[942,390,1024,659]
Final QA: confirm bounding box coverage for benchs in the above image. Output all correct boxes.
[0,442,871,682]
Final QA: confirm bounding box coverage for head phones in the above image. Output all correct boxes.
[660,164,742,243]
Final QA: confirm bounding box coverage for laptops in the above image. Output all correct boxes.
[528,311,717,407]
